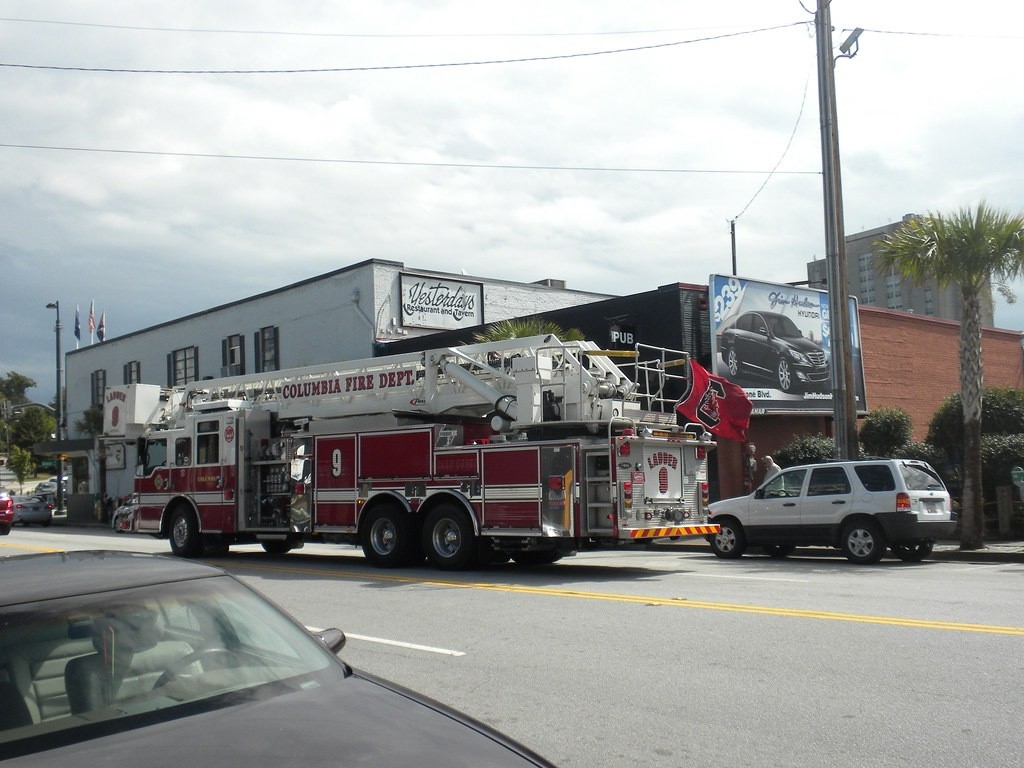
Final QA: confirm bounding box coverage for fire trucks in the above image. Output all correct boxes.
[98,333,722,572]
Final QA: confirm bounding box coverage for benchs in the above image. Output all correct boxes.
[0,614,100,724]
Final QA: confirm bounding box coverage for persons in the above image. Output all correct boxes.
[761,455,785,496]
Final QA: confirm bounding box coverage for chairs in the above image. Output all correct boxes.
[64,604,203,717]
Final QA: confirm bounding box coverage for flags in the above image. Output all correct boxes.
[96,312,104,341]
[88,300,95,333]
[74,304,81,341]
[675,357,754,443]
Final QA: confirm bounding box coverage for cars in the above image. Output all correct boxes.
[0,550,556,768]
[35,482,58,500]
[11,495,53,527]
[722,311,831,394]
[0,486,13,535]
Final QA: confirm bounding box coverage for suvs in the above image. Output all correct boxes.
[700,459,958,565]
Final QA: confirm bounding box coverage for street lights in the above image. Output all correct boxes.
[46,301,67,515]
